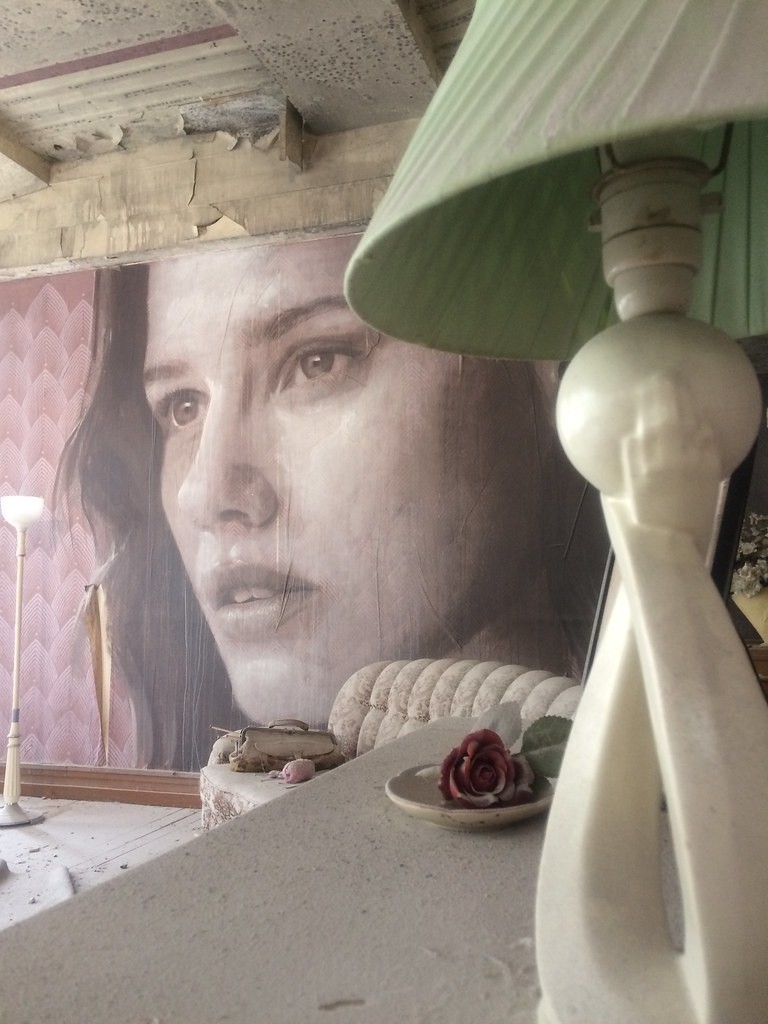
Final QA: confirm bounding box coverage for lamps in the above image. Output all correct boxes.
[0,494,43,827]
[342,0,768,1024]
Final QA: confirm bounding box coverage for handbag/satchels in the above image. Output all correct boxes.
[228,719,338,774]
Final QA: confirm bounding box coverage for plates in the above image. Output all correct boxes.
[383,764,557,831]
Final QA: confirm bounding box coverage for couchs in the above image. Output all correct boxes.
[198,659,583,834]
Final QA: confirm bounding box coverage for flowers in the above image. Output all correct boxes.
[438,716,574,807]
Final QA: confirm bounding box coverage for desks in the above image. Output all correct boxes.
[1,712,683,1023]
